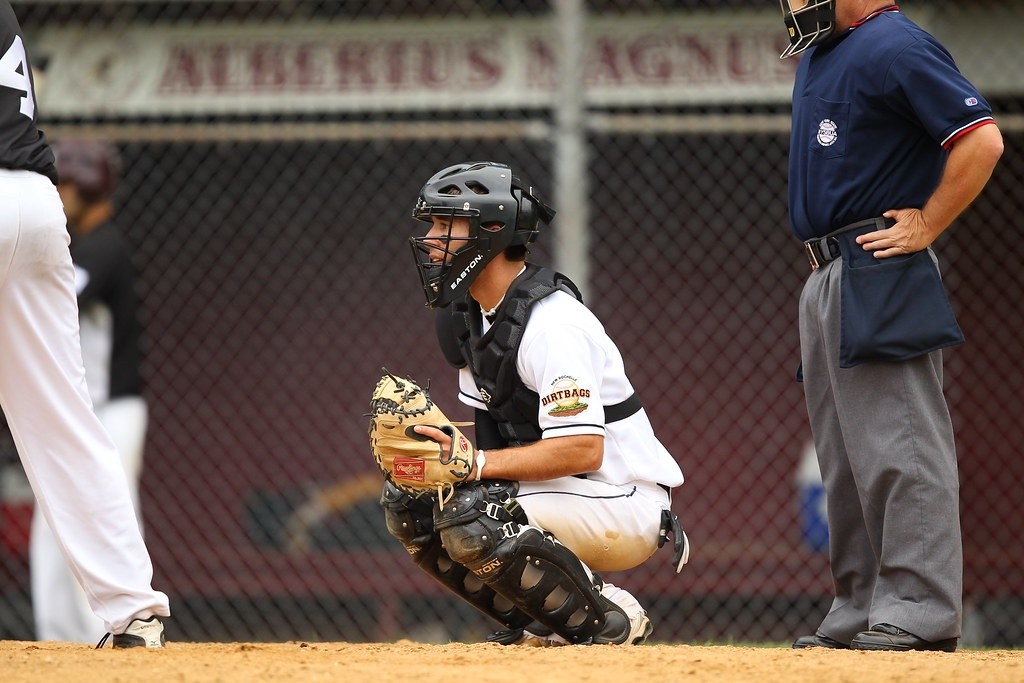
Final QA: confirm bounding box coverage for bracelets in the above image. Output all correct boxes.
[475,449,486,481]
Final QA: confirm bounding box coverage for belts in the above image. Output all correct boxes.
[804,236,841,270]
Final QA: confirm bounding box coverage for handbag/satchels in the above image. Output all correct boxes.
[840,225,967,369]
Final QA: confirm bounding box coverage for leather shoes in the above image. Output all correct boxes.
[792,634,850,649]
[851,624,958,651]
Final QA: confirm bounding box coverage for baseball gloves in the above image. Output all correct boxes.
[367,370,474,497]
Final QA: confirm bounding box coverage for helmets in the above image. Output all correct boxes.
[49,136,124,206]
[408,161,556,312]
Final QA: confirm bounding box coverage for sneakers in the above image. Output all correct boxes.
[94,614,166,650]
[493,583,653,648]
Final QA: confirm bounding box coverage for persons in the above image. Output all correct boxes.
[778,0,1005,651]
[0,0,172,649]
[27,134,151,643]
[368,160,690,649]
